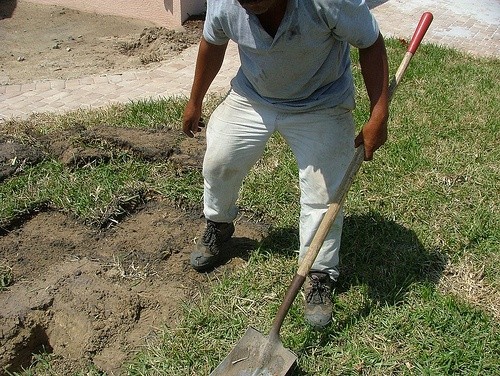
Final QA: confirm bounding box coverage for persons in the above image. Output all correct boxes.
[183,1,391,331]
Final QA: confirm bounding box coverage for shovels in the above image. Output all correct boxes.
[208,13,454,375]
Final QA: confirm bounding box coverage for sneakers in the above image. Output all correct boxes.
[302,269,338,330]
[192,220,235,272]
